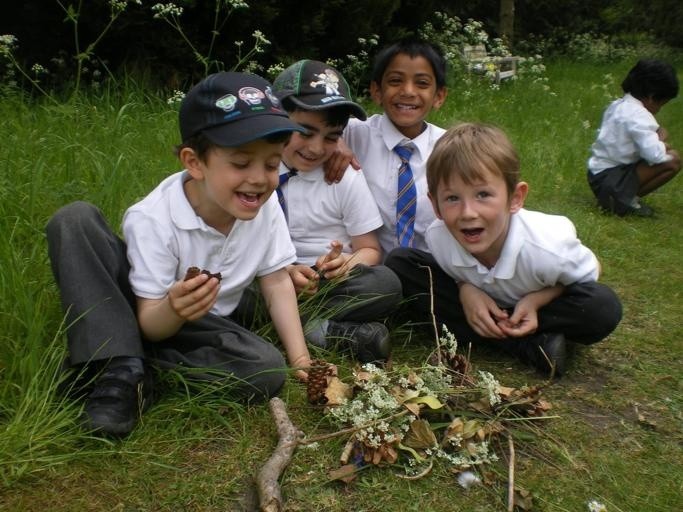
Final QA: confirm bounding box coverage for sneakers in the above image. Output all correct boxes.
[323,319,391,363]
[628,194,655,217]
[74,359,154,432]
[513,331,568,379]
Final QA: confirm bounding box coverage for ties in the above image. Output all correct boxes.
[392,146,416,249]
[276,168,295,223]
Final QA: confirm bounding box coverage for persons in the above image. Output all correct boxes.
[587,56,683,218]
[227,57,403,361]
[322,38,449,265]
[45,71,337,434]
[383,121,623,378]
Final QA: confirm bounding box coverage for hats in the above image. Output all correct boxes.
[271,59,366,121]
[178,71,307,148]
